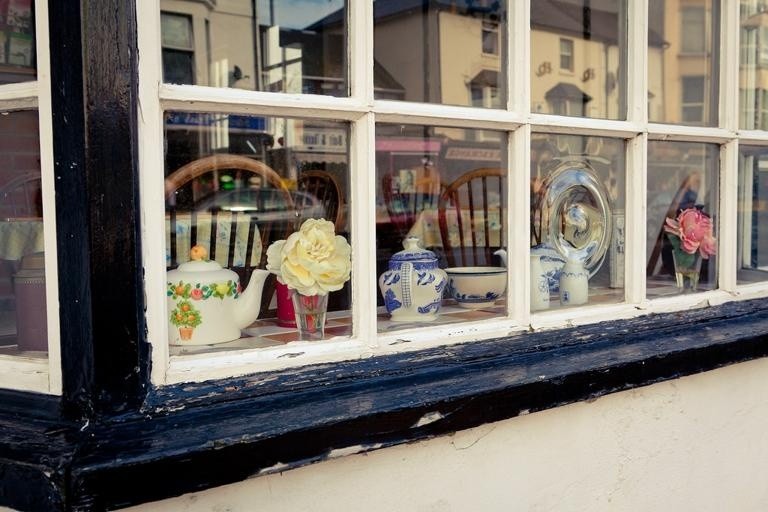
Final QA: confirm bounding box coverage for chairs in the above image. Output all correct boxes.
[163,154,296,318]
[438,168,542,268]
[295,171,345,235]
[408,176,453,225]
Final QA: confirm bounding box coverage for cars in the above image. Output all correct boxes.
[647,189,693,241]
[164,188,325,257]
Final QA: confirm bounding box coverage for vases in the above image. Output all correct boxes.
[293,290,328,337]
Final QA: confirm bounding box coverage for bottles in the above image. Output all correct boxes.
[530,255,549,310]
[13,253,47,352]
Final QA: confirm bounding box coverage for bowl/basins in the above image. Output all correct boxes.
[444,266,508,309]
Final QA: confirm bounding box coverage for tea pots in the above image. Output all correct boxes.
[165,243,270,347]
[494,235,597,295]
[379,236,449,322]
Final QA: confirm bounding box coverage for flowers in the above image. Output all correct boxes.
[664,206,718,259]
[265,218,353,295]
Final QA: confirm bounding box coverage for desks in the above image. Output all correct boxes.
[402,209,602,249]
[164,210,262,268]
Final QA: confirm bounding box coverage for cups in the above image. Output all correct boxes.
[291,292,330,333]
